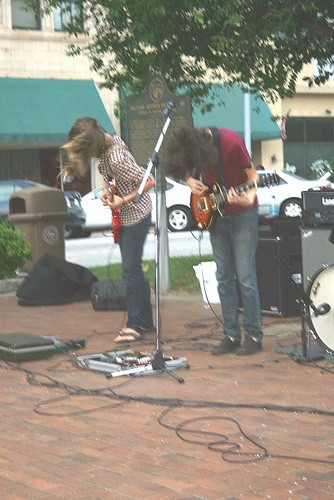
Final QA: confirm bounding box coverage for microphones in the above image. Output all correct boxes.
[161,99,175,118]
[316,303,329,316]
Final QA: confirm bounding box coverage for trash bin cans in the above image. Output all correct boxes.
[6,186,68,271]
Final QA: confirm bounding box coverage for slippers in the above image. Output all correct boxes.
[113,330,143,343]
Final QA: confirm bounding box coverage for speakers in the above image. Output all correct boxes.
[255,237,301,315]
[300,225,334,314]
[91,278,151,310]
[16,252,100,306]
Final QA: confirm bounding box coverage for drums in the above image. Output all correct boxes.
[305,264,334,358]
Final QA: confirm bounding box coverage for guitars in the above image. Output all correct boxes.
[193,173,281,231]
[112,181,119,244]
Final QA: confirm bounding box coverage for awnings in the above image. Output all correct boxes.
[0,77,115,144]
[120,80,283,146]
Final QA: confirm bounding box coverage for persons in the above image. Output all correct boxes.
[164,127,263,356]
[58,117,155,343]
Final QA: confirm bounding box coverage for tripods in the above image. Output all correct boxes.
[104,119,189,382]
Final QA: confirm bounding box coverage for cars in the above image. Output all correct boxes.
[78,176,193,232]
[255,170,334,220]
[0,180,87,239]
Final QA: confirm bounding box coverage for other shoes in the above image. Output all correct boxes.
[211,336,240,355]
[235,338,262,355]
